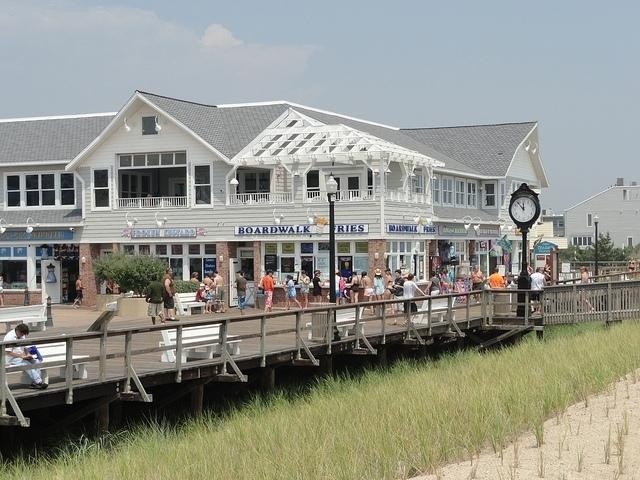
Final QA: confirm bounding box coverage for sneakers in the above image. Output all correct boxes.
[165,316,180,322]
[204,309,226,314]
[28,382,49,390]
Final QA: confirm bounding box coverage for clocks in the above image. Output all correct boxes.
[507,181,543,317]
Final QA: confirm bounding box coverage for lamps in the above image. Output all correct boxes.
[228,164,241,186]
[1,217,7,234]
[402,207,422,225]
[306,207,318,225]
[462,216,473,233]
[124,116,132,132]
[424,208,437,225]
[272,209,284,225]
[154,114,162,132]
[26,217,35,233]
[155,211,167,229]
[124,211,137,229]
[470,216,482,233]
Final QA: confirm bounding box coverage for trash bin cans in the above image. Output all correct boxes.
[255,294,265,310]
[310,302,337,343]
[494,288,510,316]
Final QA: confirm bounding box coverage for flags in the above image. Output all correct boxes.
[497,235,513,256]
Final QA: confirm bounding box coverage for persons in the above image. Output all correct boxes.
[576,267,594,314]
[145,275,166,325]
[233,269,328,316]
[162,268,180,321]
[190,268,226,315]
[628,257,636,272]
[3,324,48,389]
[72,275,84,310]
[334,263,552,327]
[106,280,120,294]
[0,272,5,308]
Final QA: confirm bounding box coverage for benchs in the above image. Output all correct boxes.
[160,322,243,364]
[306,306,366,341]
[411,294,457,324]
[0,302,49,332]
[0,333,90,389]
[174,292,206,316]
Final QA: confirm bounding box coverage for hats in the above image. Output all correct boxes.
[315,270,321,274]
[374,268,382,276]
[236,270,243,274]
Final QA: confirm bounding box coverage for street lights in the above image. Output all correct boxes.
[592,214,601,284]
[325,173,342,341]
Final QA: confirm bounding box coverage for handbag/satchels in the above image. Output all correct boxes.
[351,283,360,292]
